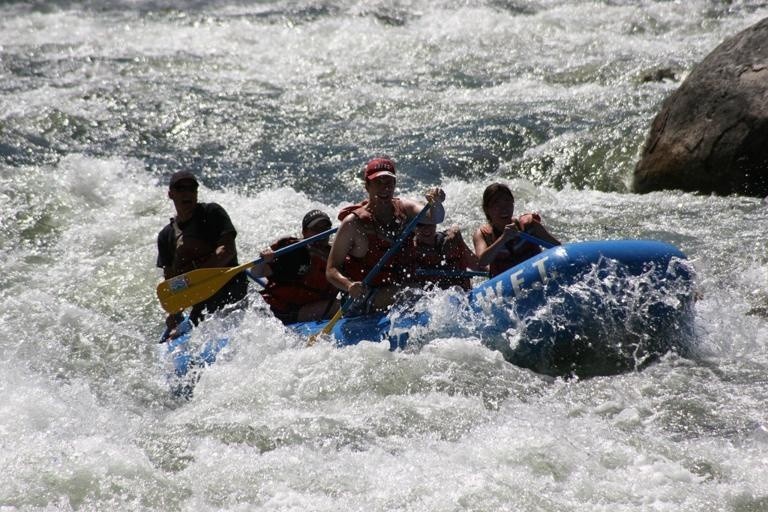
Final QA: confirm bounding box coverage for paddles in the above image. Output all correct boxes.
[155,187,400,315]
[304,201,431,346]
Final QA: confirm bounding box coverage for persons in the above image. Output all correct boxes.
[154,168,251,331]
[324,157,446,319]
[403,220,491,293]
[467,182,562,280]
[249,209,344,326]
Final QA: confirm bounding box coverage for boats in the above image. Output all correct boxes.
[156,238,702,399]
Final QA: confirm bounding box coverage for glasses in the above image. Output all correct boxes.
[173,187,197,192]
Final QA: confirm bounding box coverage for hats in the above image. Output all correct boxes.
[365,160,396,180]
[303,210,332,231]
[170,172,198,186]
